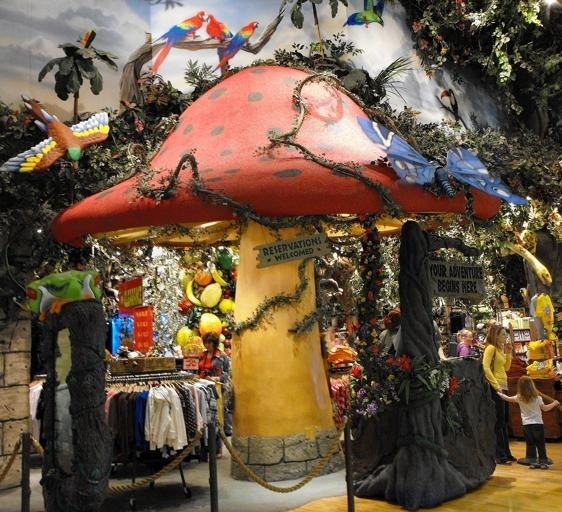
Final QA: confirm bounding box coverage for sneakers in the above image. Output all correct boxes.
[216,447,222,458]
[495,456,549,469]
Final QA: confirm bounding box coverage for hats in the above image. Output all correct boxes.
[388,306,401,318]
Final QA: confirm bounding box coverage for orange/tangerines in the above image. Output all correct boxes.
[219,251,234,270]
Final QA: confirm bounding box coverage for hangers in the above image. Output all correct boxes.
[105,371,198,391]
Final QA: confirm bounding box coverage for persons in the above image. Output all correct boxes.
[376,306,403,361]
[197,331,232,378]
[495,374,560,467]
[481,323,517,466]
[453,328,475,358]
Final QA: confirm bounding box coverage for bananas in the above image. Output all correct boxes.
[186,279,201,306]
[211,263,229,287]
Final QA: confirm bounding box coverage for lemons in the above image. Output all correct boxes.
[199,313,222,340]
[200,283,222,308]
[176,325,199,349]
[218,299,235,315]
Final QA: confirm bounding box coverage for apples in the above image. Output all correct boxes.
[178,299,193,316]
[194,269,213,286]
